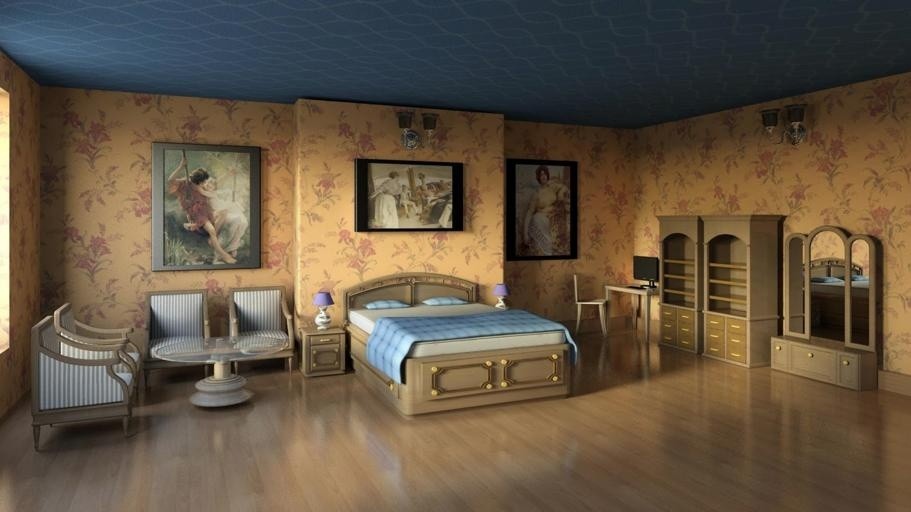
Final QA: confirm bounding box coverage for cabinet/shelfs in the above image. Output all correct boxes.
[770,336,877,391]
[656,215,703,354]
[699,215,784,368]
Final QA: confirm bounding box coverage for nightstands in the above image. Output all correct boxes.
[299,328,347,378]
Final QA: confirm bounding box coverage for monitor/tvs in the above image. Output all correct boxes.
[633,255,659,289]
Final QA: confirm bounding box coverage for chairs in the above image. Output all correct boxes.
[31,315,135,451]
[53,302,141,400]
[227,286,295,375]
[573,275,607,338]
[143,288,211,379]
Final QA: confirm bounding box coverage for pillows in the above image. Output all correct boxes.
[811,276,842,282]
[851,275,867,280]
[422,296,467,305]
[361,300,409,310]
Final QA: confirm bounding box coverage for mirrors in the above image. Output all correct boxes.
[785,225,877,353]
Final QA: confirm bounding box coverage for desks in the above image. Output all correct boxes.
[603,283,660,342]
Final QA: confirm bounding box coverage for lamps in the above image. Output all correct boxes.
[315,291,334,330]
[397,111,422,149]
[493,284,510,310]
[422,112,439,145]
[760,102,806,146]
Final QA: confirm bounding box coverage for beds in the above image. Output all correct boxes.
[342,272,579,415]
[801,257,869,316]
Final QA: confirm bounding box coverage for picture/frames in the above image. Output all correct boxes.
[354,158,464,232]
[506,158,578,260]
[149,142,261,272]
